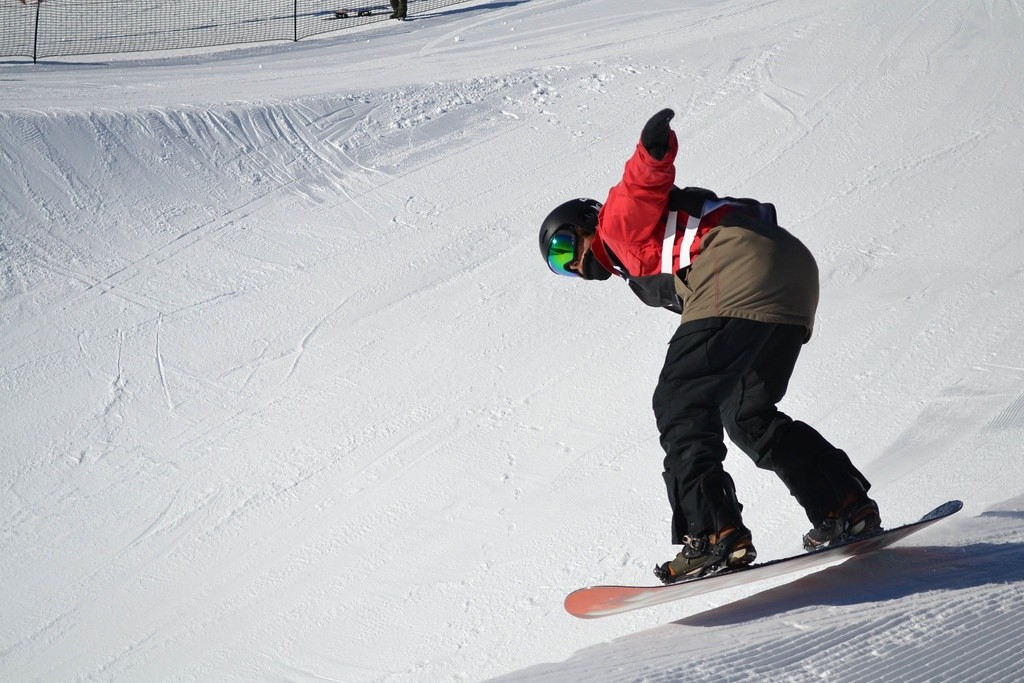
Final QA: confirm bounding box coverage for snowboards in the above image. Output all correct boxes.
[564,498,965,623]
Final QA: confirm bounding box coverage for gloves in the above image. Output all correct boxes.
[641,108,675,160]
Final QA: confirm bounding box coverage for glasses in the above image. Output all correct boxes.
[546,227,580,278]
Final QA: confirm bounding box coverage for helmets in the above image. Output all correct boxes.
[539,199,603,275]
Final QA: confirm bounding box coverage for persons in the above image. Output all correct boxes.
[540,108,881,582]
[389,0,407,20]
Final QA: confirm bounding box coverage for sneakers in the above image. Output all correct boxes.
[801,496,880,551]
[658,523,756,584]
